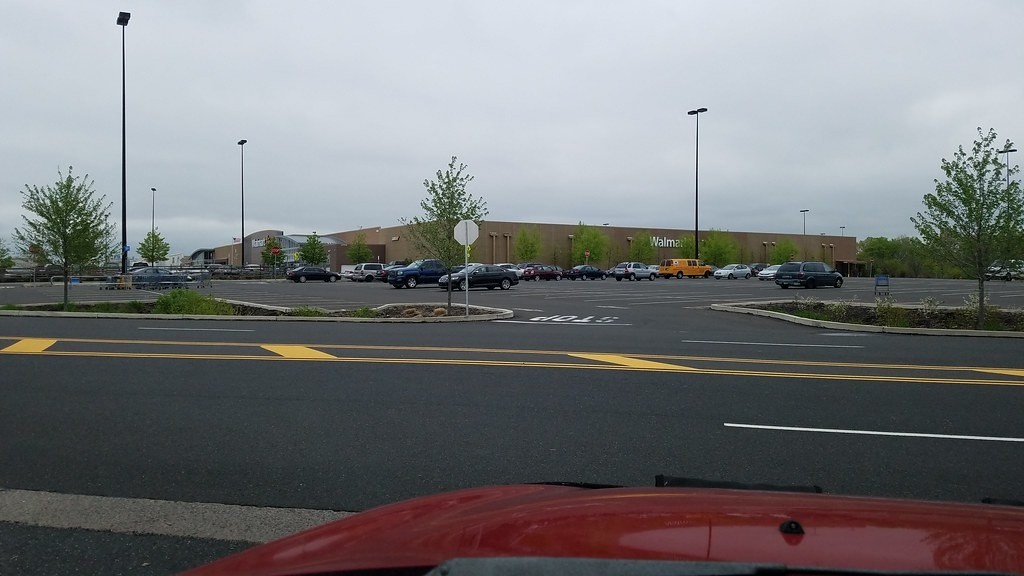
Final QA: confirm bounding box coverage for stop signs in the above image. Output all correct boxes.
[585,250,590,256]
[271,246,281,255]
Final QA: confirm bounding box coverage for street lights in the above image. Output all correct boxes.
[151,187,156,268]
[236,139,249,268]
[840,226,845,237]
[800,209,810,236]
[998,149,1018,212]
[115,10,133,275]
[104,214,108,261]
[687,108,710,260]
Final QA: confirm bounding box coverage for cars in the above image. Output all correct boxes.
[438,264,520,292]
[757,264,782,281]
[286,266,342,284]
[984,259,1024,282]
[605,266,617,278]
[568,265,610,281]
[374,265,407,284]
[344,270,354,280]
[168,475,1024,576]
[713,263,752,280]
[2,251,294,288]
[468,261,572,282]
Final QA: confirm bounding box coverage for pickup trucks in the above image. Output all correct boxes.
[387,258,465,289]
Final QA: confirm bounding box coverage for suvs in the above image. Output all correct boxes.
[615,262,660,282]
[352,262,391,283]
[774,259,844,290]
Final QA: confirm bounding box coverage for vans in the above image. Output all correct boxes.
[658,259,711,279]
